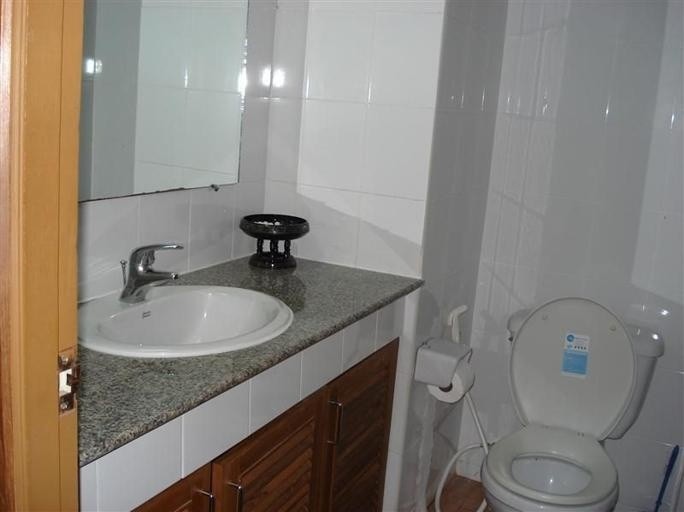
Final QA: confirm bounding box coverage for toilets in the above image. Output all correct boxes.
[474,296,663,511]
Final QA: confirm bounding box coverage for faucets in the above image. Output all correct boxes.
[117,243,182,302]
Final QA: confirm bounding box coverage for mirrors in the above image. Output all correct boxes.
[78,0,253,204]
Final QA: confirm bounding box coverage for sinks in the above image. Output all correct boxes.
[75,282,296,361]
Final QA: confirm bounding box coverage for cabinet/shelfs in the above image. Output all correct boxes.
[318,337,400,511]
[131,389,318,511]
